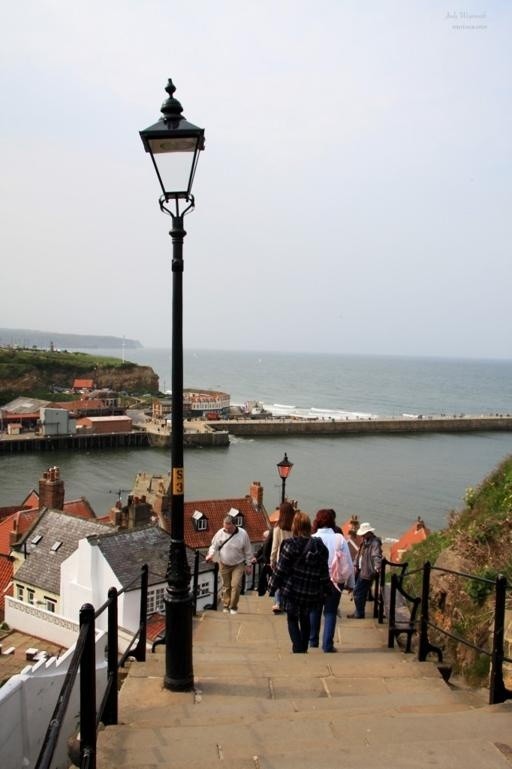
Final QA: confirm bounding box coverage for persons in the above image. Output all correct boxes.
[252,501,385,653]
[205,516,253,614]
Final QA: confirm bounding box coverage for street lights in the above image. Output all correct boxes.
[137,78,206,691]
[276,450,294,504]
